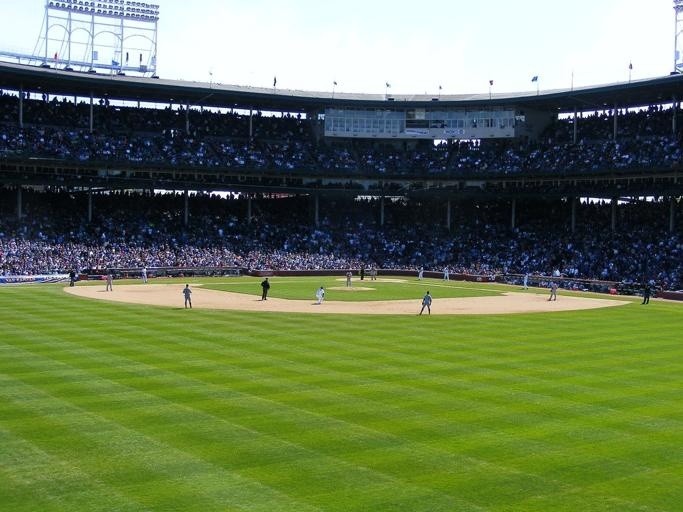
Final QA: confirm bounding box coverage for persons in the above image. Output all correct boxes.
[260,278,270,300]
[1,91,384,291]
[385,91,682,304]
[183,284,193,309]
[420,291,432,314]
[315,286,324,303]
